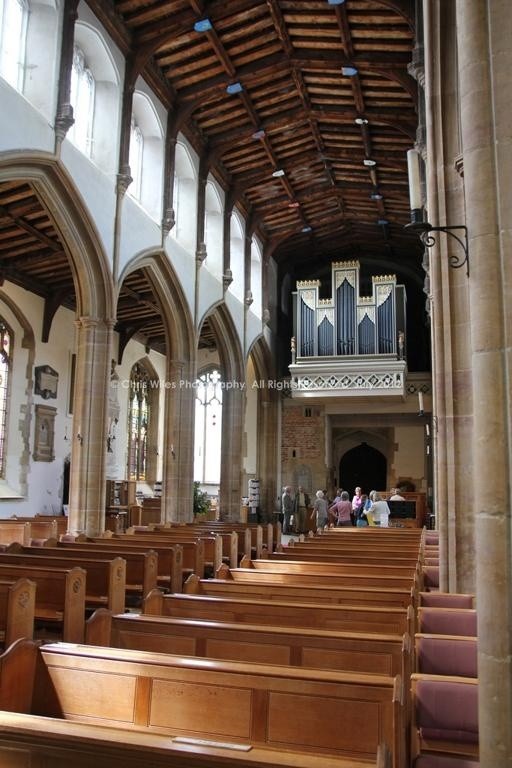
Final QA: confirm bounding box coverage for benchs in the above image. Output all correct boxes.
[0,509,479,767]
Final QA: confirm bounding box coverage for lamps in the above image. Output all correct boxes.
[154,481,162,497]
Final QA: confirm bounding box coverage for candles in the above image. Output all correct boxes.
[171,443,173,452]
[418,391,424,413]
[78,425,81,434]
[406,148,423,210]
[426,444,429,455]
[425,423,429,435]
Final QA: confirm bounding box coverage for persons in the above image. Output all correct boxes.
[276,485,405,537]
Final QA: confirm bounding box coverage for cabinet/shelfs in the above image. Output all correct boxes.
[106,478,137,509]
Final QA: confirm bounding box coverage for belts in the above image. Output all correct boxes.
[299,506,306,508]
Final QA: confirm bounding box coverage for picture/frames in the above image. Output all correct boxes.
[67,350,78,418]
